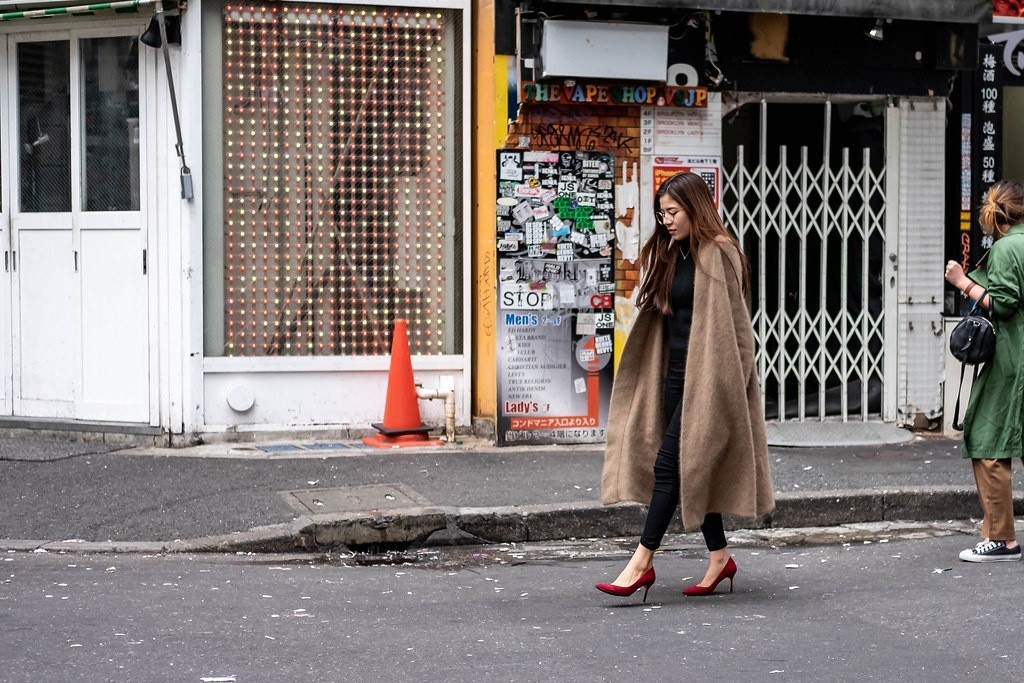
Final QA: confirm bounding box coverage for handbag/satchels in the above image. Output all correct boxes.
[950,290,996,365]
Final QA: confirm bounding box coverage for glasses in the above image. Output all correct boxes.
[656,208,684,225]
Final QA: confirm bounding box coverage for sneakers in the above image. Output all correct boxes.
[959,538,1021,563]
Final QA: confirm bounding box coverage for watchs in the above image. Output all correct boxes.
[960,282,976,298]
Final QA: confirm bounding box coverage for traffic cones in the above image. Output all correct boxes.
[362,319,444,448]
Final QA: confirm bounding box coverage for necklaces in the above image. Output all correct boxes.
[680,246,692,259]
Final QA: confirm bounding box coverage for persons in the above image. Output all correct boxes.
[595,172,776,602]
[945,179,1024,563]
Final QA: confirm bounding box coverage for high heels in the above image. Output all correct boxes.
[682,557,737,596]
[595,566,655,602]
